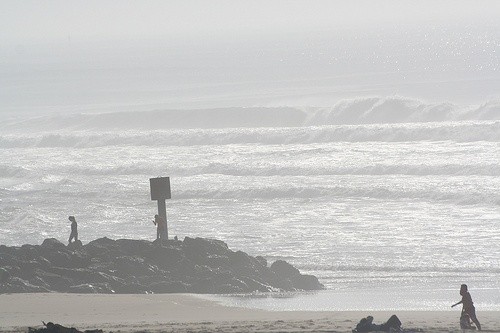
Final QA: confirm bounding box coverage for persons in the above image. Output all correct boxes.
[47,322,103,333]
[152,214,166,238]
[68,216,78,245]
[358,314,408,333]
[451,284,480,331]
